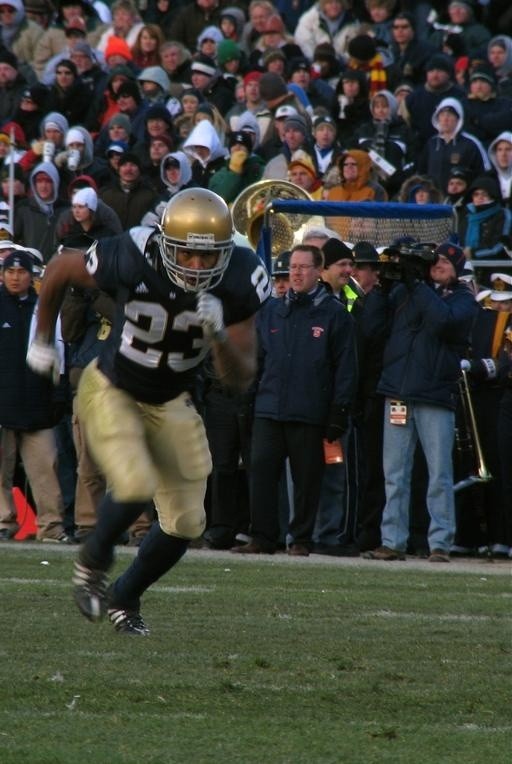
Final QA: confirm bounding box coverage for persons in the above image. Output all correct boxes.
[26,187,274,636]
[0,0,512,562]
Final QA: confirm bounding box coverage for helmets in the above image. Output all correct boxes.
[158,187,235,292]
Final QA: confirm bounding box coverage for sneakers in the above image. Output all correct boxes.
[190,533,361,557]
[102,582,150,635]
[71,559,107,620]
[428,549,450,562]
[38,530,149,547]
[450,538,477,557]
[490,536,510,557]
[370,546,405,560]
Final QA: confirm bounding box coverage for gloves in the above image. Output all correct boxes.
[325,412,350,444]
[27,343,62,385]
[198,292,228,342]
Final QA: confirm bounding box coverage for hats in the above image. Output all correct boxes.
[4,252,34,274]
[353,241,381,265]
[437,244,466,274]
[0,13,496,213]
[271,252,292,275]
[322,238,350,269]
[385,236,411,255]
